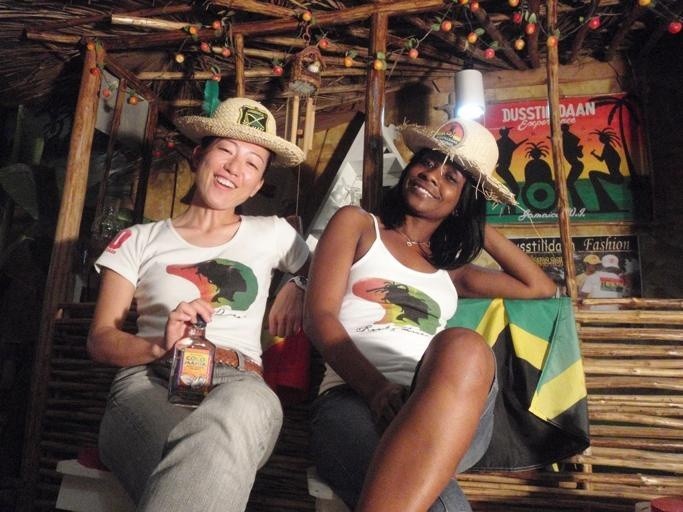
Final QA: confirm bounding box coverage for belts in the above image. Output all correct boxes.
[163,346,263,377]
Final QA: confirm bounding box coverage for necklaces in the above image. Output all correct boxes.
[394,228,429,247]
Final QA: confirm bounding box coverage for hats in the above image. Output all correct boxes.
[172,97,305,169]
[583,255,601,265]
[401,117,517,208]
[602,255,620,269]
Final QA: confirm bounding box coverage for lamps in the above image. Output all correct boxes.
[452,68,486,122]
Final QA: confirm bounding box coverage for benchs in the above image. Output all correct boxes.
[32,295,683,512]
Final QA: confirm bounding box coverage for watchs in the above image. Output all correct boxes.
[286,275,306,290]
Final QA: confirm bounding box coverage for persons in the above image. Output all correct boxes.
[302,117,558,512]
[82,97,314,512]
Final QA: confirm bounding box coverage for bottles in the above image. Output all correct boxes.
[85,207,120,268]
[167,314,216,403]
